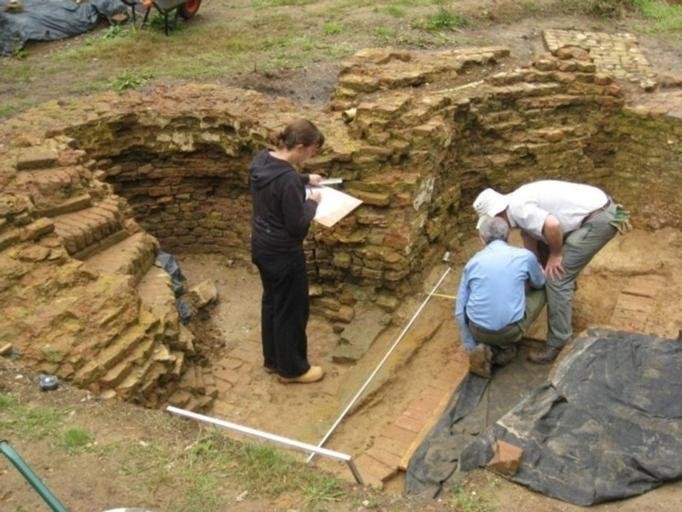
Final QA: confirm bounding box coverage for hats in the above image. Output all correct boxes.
[472,186,512,231]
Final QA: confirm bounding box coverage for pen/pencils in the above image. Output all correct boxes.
[308,183,314,195]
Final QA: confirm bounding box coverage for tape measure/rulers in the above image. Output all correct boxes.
[36,372,58,392]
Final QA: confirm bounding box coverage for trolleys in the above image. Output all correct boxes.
[120,0,200,34]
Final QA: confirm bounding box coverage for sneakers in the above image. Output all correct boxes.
[470,343,517,381]
[526,346,562,366]
[263,364,324,385]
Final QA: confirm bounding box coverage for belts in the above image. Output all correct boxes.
[580,193,612,229]
[468,320,523,336]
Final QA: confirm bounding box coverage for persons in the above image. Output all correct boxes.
[452,215,546,379]
[471,178,625,365]
[251,118,326,383]
